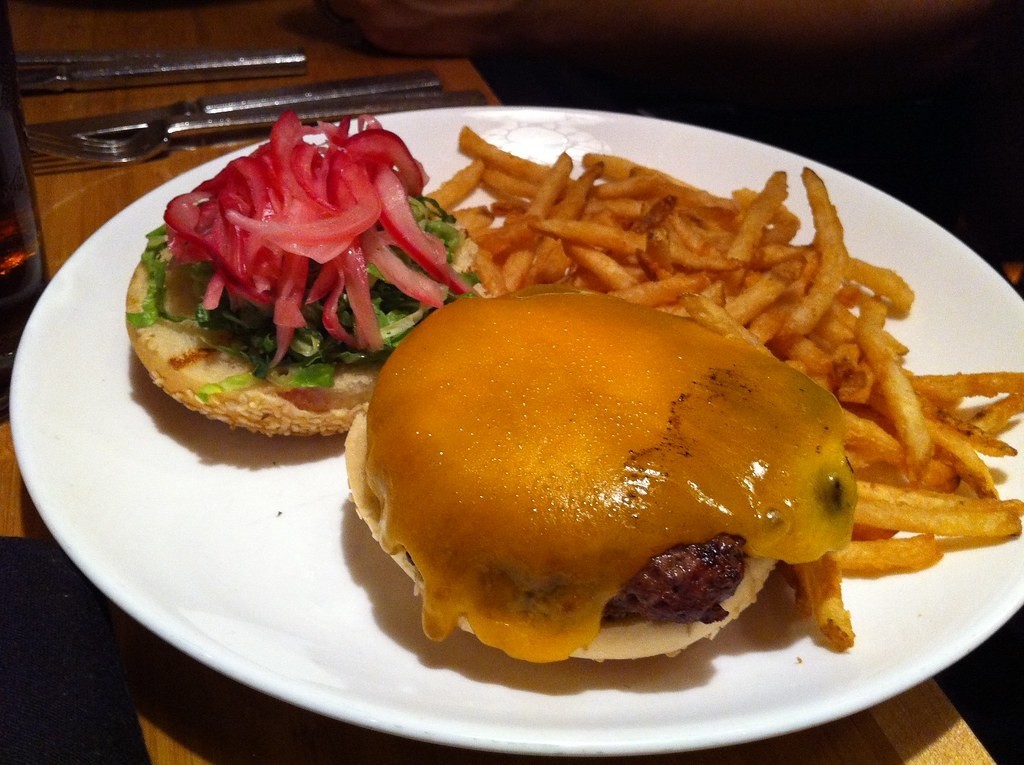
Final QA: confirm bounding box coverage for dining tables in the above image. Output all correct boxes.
[0,2,997,765]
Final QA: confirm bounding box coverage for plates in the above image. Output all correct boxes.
[9,106,1024,757]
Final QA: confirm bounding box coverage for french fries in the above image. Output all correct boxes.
[430,129,1024,648]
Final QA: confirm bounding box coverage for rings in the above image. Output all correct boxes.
[319,0,350,25]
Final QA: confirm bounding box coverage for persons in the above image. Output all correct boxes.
[319,0,996,61]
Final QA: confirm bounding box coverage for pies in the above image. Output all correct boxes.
[128,245,361,435]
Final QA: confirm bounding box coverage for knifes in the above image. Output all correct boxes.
[21,66,442,141]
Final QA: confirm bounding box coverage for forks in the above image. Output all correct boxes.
[24,90,488,164]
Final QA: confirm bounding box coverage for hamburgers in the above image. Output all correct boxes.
[345,287,857,662]
